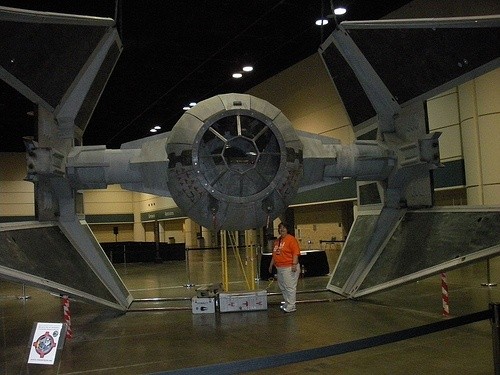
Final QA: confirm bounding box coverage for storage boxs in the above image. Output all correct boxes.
[219,290,267,312]
[192,296,215,314]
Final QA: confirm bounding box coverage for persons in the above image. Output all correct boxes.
[268,221,300,313]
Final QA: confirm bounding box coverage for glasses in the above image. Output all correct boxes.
[280,229,286,231]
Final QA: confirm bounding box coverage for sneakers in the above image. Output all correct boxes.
[280,305,296,312]
